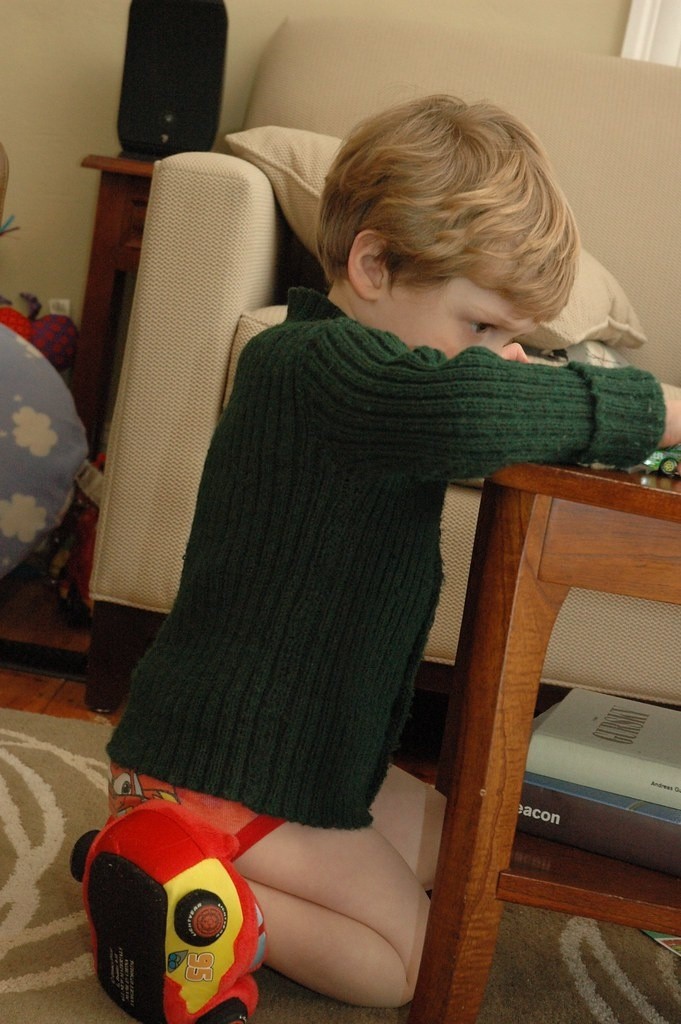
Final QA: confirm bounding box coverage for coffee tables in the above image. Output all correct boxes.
[408,460,681,1023]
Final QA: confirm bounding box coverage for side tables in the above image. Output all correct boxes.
[74,151,159,451]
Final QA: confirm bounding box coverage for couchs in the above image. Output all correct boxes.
[86,2,680,715]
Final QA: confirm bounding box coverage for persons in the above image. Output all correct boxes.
[70,93,681,1010]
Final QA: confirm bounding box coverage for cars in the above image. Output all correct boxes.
[642,442,681,477]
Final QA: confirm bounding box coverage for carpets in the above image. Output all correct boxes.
[0,706,680,1023]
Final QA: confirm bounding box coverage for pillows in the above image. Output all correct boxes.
[225,124,644,355]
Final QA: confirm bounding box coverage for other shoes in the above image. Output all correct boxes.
[68,800,269,1024]
